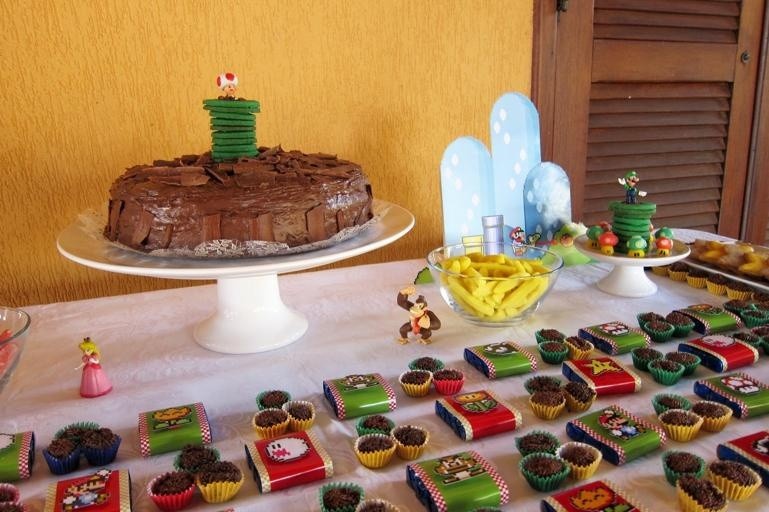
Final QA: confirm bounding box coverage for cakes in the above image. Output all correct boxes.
[585,171,672,257]
[102,70,375,255]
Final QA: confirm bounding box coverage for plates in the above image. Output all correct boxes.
[663,225,769,289]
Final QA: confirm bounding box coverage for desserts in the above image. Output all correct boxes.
[0,240,768,512]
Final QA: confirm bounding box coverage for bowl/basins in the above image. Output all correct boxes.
[424,244,564,327]
[1,303,31,392]
